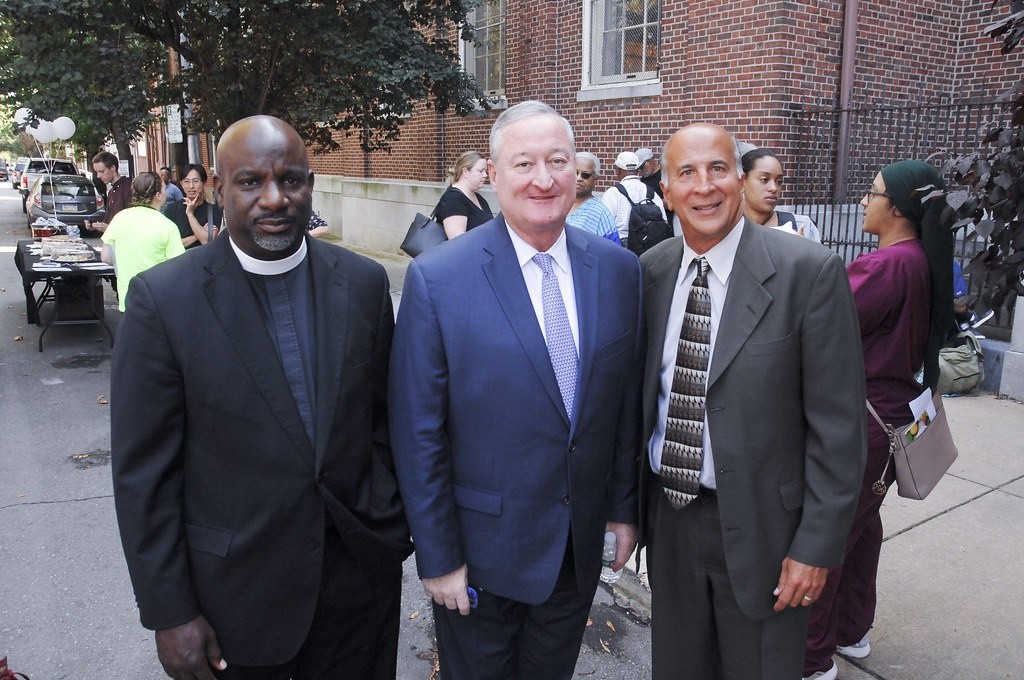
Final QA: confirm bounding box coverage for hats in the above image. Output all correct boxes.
[612,151,640,170]
[635,148,653,166]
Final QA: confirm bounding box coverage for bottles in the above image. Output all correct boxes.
[600,531,624,583]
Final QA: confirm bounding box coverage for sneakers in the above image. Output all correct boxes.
[969,309,995,328]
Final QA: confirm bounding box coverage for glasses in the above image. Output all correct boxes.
[183,178,202,185]
[866,184,892,203]
[577,171,597,179]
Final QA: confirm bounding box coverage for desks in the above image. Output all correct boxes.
[14,239,118,352]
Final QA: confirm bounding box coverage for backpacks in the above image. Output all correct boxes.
[616,183,673,257]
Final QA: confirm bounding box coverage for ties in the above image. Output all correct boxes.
[532,253,579,424]
[660,255,711,511]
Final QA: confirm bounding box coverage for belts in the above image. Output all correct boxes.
[621,238,628,242]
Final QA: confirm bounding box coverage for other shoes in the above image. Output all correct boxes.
[802,662,838,680]
[837,633,870,658]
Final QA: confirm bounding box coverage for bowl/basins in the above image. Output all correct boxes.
[36,230,54,236]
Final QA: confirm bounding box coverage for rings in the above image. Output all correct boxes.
[804,596,813,602]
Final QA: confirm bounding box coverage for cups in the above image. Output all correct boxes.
[43,245,52,254]
[51,246,60,260]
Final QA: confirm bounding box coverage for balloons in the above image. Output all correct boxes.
[15,108,57,143]
[53,117,76,140]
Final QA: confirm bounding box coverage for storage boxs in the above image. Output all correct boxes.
[31,224,66,237]
[54,280,105,319]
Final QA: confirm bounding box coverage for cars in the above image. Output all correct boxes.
[0,155,29,190]
[18,172,105,239]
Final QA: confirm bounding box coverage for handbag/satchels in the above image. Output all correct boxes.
[937,330,986,396]
[872,388,958,500]
[400,189,471,259]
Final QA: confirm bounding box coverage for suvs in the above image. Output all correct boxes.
[20,158,87,214]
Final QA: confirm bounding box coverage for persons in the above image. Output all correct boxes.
[740,147,822,247]
[307,208,330,238]
[637,120,869,680]
[163,164,223,250]
[601,151,669,249]
[801,159,957,680]
[160,165,187,197]
[944,259,972,347]
[435,151,494,240]
[108,114,415,680]
[100,171,187,313]
[84,151,133,302]
[388,97,644,680]
[159,169,183,214]
[564,151,622,248]
[634,147,675,224]
